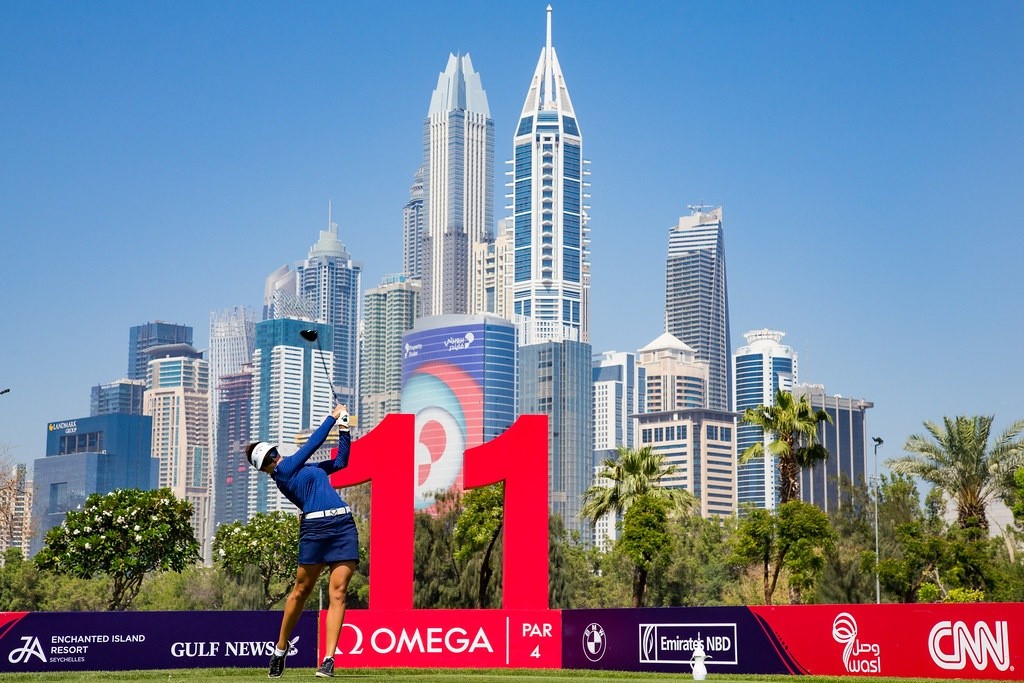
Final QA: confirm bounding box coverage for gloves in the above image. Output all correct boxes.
[334,411,350,428]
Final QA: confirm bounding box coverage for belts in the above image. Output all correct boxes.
[305,506,351,519]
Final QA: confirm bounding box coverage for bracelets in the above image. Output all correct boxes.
[340,429,349,431]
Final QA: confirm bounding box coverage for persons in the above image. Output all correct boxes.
[246,405,360,679]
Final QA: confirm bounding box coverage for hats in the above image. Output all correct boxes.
[252,441,278,471]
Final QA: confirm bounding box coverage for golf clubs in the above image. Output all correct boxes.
[300,329,349,423]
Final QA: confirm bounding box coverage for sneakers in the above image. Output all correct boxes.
[316,657,334,677]
[268,640,291,678]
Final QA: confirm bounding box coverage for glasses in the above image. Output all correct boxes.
[260,447,278,470]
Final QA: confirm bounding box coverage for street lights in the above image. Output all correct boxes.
[871,436,889,606]
